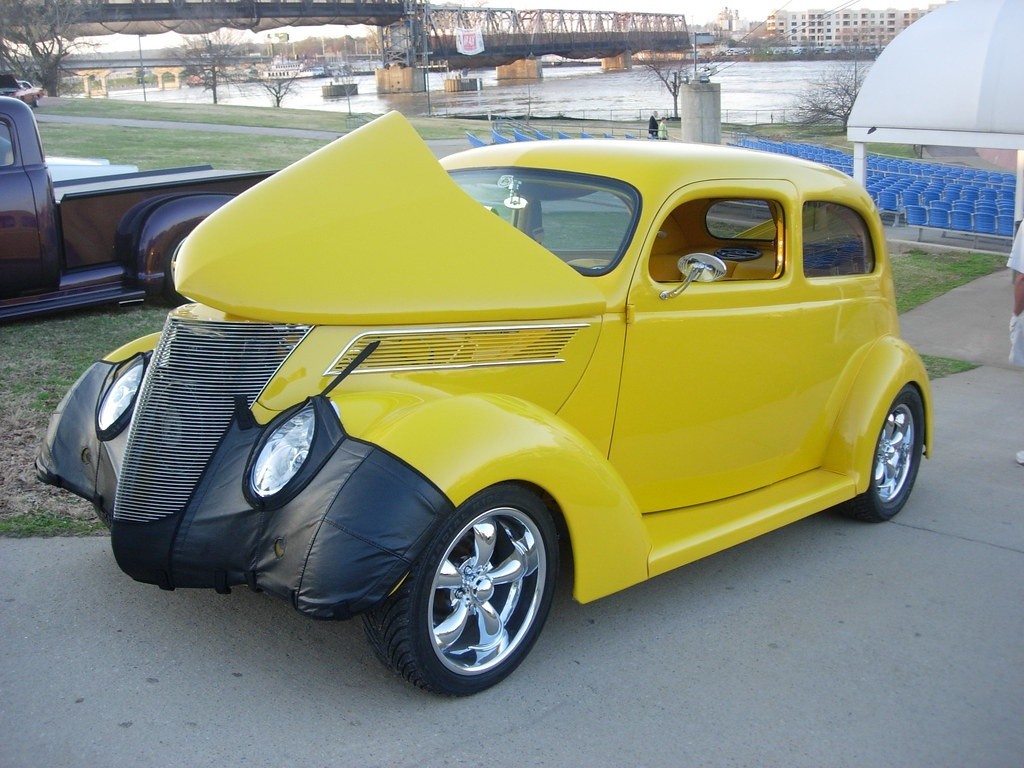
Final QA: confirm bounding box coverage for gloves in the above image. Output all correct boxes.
[1009,316,1019,333]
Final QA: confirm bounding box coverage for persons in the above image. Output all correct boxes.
[648,110,669,140]
[1007,222,1024,466]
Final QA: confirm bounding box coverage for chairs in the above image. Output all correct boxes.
[466,128,658,149]
[727,135,1017,235]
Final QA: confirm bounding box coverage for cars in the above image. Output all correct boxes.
[716,47,880,56]
[27,108,936,698]
[0,74,45,107]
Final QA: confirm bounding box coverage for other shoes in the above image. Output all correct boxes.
[1016,450,1024,465]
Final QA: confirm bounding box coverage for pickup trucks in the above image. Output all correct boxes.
[0,96,292,327]
